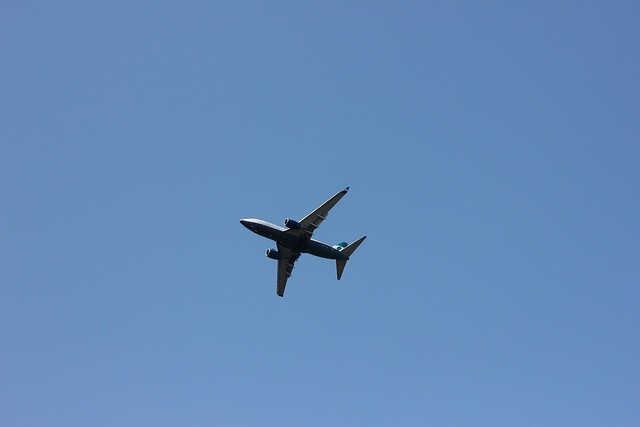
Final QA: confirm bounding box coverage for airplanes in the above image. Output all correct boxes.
[239,187,367,297]
